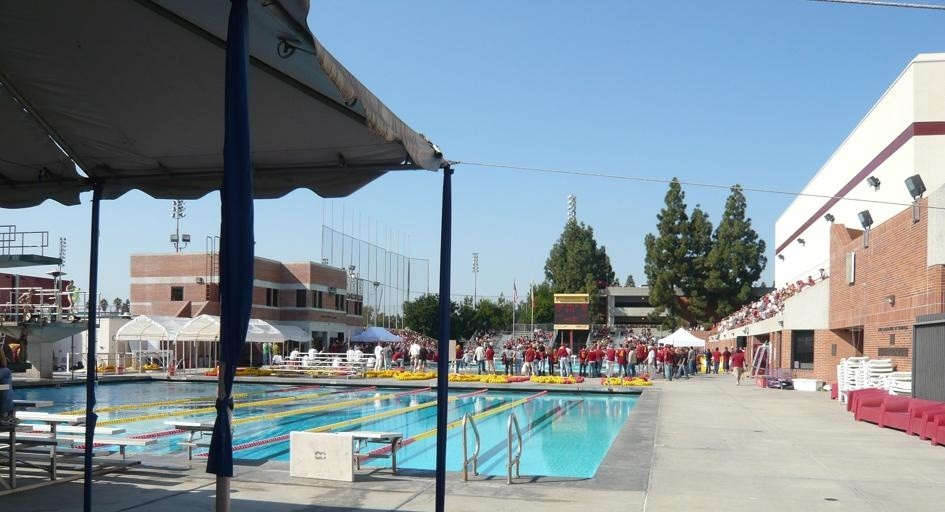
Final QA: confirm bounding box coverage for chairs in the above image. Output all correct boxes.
[846,388,945,446]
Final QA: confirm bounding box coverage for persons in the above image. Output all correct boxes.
[4,342,25,368]
[0,346,20,426]
[65,279,81,312]
[18,288,35,322]
[705,346,747,384]
[716,268,827,334]
[16,333,27,363]
[261,328,437,373]
[556,325,706,382]
[454,326,555,376]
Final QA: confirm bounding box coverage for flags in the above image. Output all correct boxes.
[514,282,534,307]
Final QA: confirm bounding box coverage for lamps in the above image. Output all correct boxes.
[824,173,926,230]
